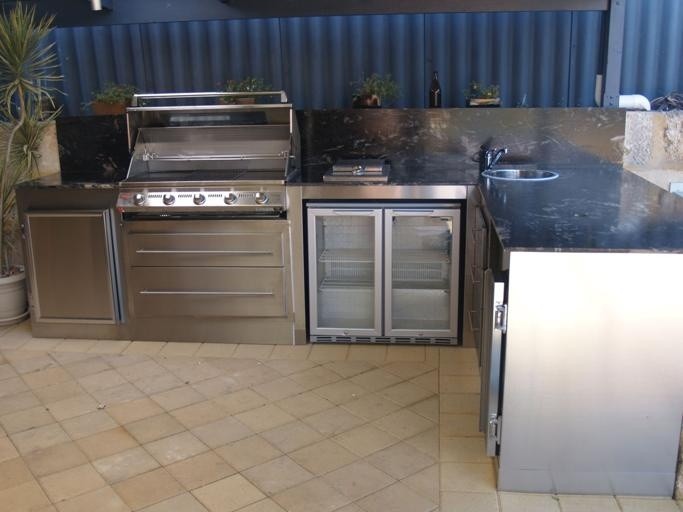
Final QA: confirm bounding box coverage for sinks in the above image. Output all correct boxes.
[323,164,391,183]
[481,168,560,181]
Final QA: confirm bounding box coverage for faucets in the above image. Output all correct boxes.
[352,138,367,159]
[484,147,512,171]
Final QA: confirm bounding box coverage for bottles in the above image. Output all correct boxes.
[429,71,442,108]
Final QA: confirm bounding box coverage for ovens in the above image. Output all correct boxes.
[115,185,295,319]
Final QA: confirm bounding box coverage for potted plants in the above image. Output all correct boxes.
[463,82,502,107]
[90,80,144,115]
[0,0,70,326]
[348,73,399,108]
[223,77,271,104]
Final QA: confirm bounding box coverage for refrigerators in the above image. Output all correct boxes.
[305,202,462,346]
